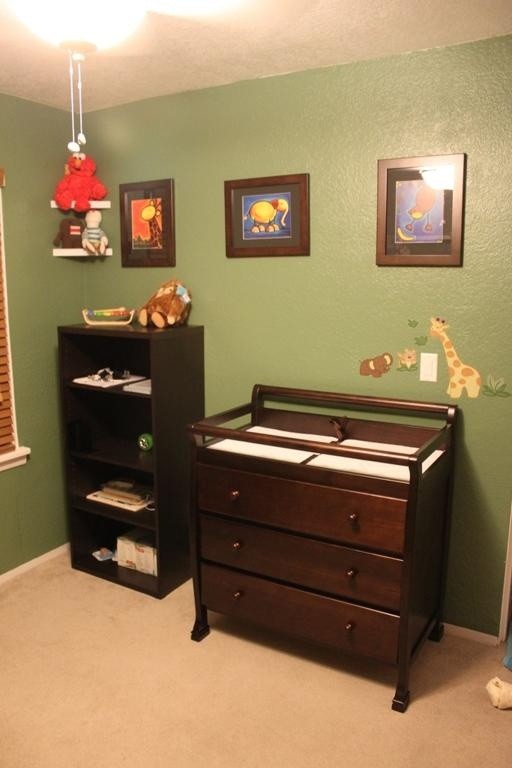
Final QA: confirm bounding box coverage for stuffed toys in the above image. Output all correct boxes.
[80,209,109,256]
[54,152,108,213]
[137,276,194,328]
[53,218,87,247]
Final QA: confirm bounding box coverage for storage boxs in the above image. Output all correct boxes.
[116,527,159,576]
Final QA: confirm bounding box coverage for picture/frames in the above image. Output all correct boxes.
[224,172,310,258]
[375,152,467,266]
[119,177,176,268]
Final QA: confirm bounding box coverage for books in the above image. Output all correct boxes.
[86,477,155,513]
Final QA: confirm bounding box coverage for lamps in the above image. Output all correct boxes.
[66,49,87,153]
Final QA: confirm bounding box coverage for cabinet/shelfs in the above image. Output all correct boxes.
[184,381,457,712]
[50,200,113,257]
[56,322,206,600]
